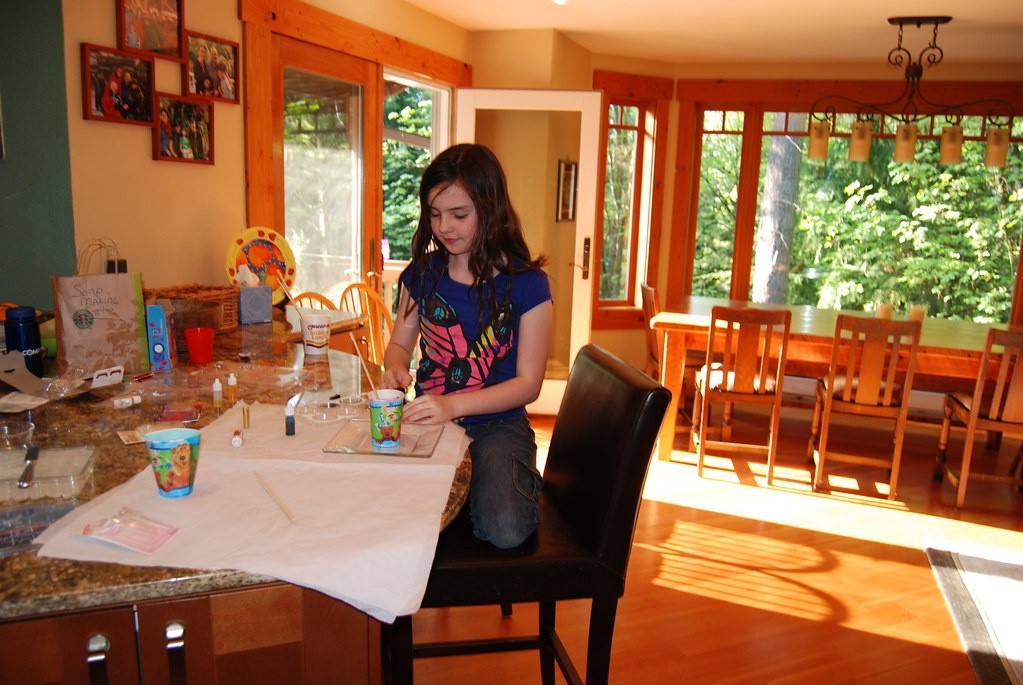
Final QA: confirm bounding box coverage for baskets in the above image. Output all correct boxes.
[143,282,238,334]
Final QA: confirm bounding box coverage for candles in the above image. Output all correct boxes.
[910,305,925,323]
[876,301,892,321]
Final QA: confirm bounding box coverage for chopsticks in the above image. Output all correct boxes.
[275,270,304,319]
[349,332,379,400]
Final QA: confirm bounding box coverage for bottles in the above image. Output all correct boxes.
[4,305,45,378]
[212,378,223,408]
[110,395,142,410]
[231,428,243,447]
[227,372,238,403]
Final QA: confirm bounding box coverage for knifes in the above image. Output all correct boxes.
[17,443,40,490]
[285,391,303,436]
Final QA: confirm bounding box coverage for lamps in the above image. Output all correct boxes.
[809,15,1017,168]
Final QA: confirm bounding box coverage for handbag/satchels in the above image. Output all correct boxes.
[51,235,151,382]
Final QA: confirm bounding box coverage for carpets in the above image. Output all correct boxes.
[924,539,1023,685]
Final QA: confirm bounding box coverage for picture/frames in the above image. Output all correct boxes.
[114,0,186,62]
[79,42,156,127]
[181,29,241,104]
[556,160,577,221]
[153,91,215,165]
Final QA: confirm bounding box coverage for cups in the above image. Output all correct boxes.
[367,388,404,449]
[184,327,215,365]
[143,428,202,498]
[299,314,331,355]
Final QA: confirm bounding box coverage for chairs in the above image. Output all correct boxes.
[380,344,671,685]
[808,315,922,501]
[933,329,1023,509]
[689,306,791,485]
[641,283,720,427]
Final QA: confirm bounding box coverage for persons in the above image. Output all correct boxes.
[379,142,552,549]
[91,7,236,162]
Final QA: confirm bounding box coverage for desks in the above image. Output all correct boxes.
[650,295,1023,461]
[0,334,471,685]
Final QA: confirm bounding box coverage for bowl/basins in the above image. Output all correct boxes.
[0,420,35,451]
